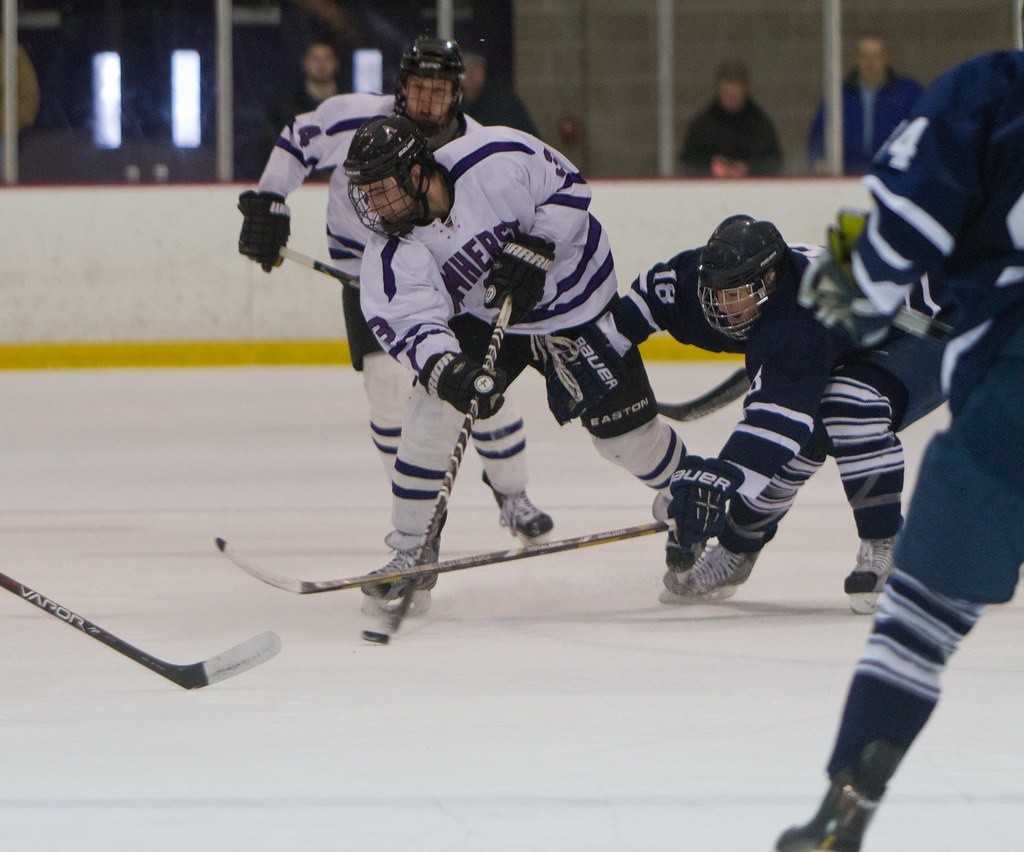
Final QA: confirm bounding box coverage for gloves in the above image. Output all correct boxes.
[797,217,898,347]
[418,354,505,420]
[236,189,289,271]
[665,454,746,543]
[482,231,558,327]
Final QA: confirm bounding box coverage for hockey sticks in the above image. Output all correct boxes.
[810,271,953,346]
[350,297,512,633]
[210,516,677,598]
[0,575,286,694]
[278,246,756,423]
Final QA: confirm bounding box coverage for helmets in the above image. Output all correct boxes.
[392,41,465,135]
[694,212,791,340]
[343,115,432,236]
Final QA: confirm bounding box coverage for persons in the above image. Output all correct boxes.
[239,36,555,552]
[621,216,945,614]
[0,34,40,135]
[456,43,541,139]
[345,113,688,646]
[776,50,1024,851]
[808,38,922,172]
[684,64,779,177]
[271,43,348,181]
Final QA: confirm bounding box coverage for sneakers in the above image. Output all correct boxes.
[777,748,875,852]
[360,514,448,621]
[479,470,553,547]
[845,528,902,616]
[658,523,777,605]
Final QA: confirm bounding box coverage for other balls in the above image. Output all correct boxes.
[360,630,390,645]
